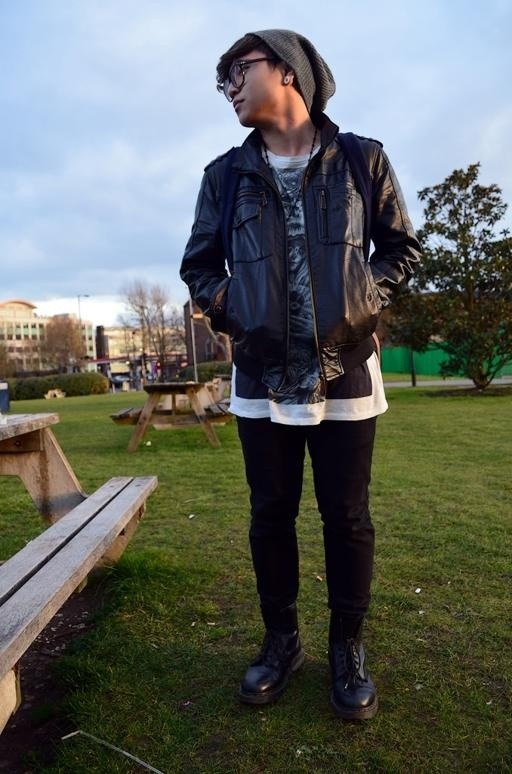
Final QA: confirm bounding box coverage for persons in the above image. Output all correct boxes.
[127,362,137,389]
[153,361,161,383]
[106,365,115,393]
[178,26,421,721]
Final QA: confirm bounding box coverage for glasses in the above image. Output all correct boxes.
[224,57,282,103]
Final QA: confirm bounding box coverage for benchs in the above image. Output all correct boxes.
[109,402,235,453]
[0,475,162,745]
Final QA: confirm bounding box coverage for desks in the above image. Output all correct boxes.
[126,381,223,451]
[0,411,148,578]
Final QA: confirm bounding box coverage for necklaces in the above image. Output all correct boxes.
[265,127,318,221]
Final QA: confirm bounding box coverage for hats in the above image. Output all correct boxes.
[245,29,336,114]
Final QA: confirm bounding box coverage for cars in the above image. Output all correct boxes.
[112,376,129,386]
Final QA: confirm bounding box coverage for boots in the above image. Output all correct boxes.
[239,602,304,704]
[326,613,378,720]
